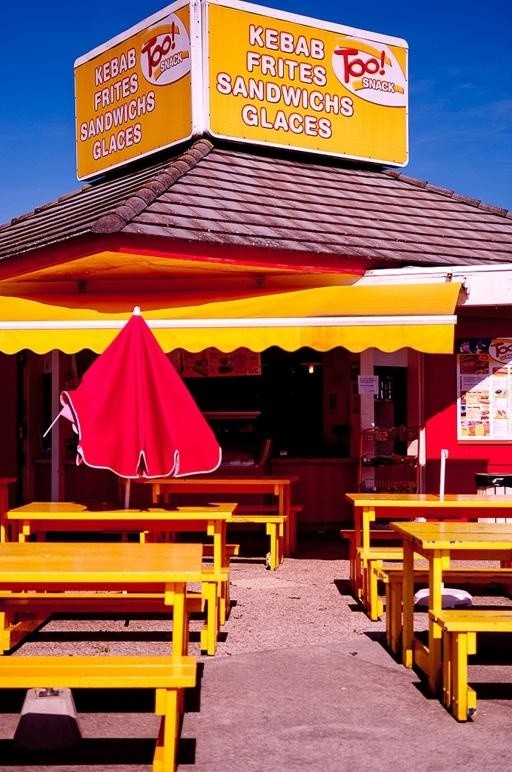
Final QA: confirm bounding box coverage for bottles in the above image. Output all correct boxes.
[373,375,395,399]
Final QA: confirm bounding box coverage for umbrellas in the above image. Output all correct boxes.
[43,303,224,508]
